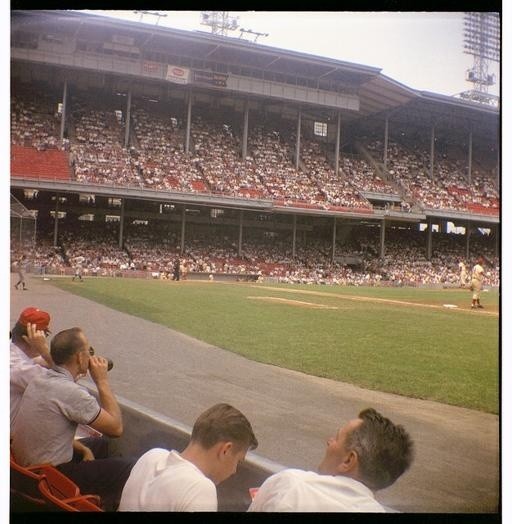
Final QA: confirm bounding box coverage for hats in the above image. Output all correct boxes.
[19,308,52,334]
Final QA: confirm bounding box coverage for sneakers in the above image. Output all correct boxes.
[15,285,18,289]
[23,288,27,290]
[471,304,483,309]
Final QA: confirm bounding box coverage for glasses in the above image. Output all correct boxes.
[82,347,94,356]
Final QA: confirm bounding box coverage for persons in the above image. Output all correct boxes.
[466,274,484,310]
[11,79,499,291]
[10,326,133,517]
[10,305,57,449]
[248,405,417,515]
[116,401,259,516]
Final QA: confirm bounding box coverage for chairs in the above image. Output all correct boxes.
[11,453,105,511]
